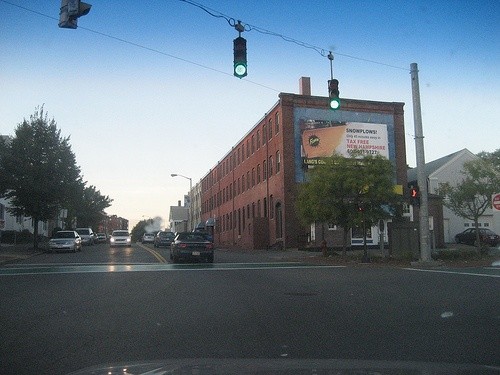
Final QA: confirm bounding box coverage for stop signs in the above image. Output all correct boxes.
[493,195,500,210]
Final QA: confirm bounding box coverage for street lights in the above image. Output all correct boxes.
[171,174,192,231]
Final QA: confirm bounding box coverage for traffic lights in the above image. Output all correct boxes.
[410,186,419,204]
[328,79,340,110]
[59,0,92,29]
[233,36,248,78]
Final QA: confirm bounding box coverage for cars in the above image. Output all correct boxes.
[93,232,107,243]
[169,233,214,263]
[108,230,133,247]
[48,230,83,253]
[455,227,500,246]
[75,228,94,246]
[154,231,175,246]
[142,232,156,244]
